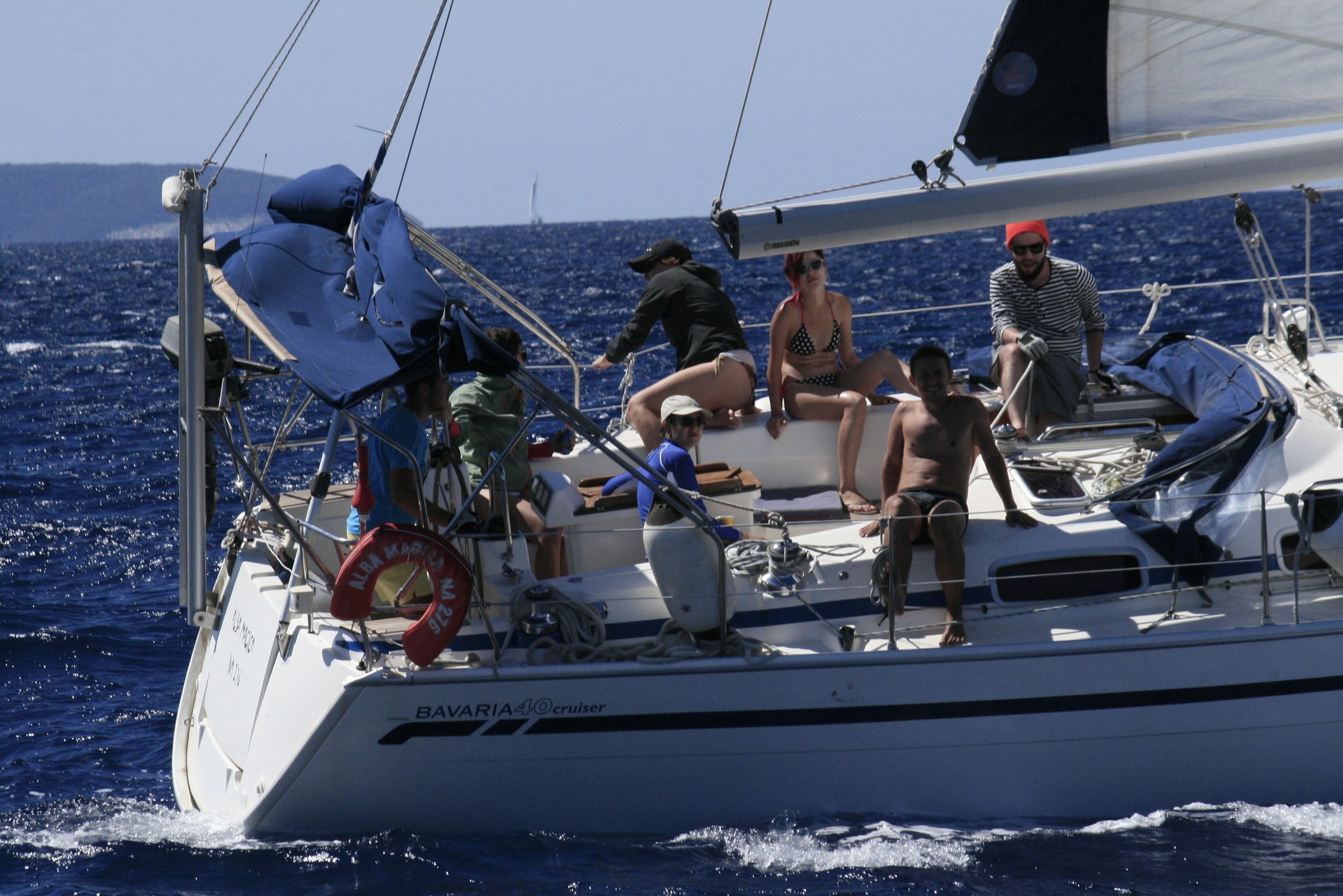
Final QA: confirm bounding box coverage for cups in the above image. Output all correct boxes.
[716,515,733,544]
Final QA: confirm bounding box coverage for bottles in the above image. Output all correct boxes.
[557,424,575,454]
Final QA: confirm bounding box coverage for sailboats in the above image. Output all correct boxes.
[160,0,1343,843]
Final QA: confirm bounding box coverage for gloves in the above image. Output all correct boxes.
[1015,330,1049,361]
[1088,363,1124,395]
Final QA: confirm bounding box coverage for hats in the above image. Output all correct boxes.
[1005,219,1050,253]
[660,395,713,425]
[628,239,692,274]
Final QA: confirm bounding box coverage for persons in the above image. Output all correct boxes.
[601,394,770,547]
[345,326,608,620]
[592,239,762,456]
[765,249,957,515]
[859,346,1038,647]
[989,219,1121,446]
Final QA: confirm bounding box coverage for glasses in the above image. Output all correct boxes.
[512,349,528,361]
[674,415,706,429]
[1010,240,1046,255]
[796,258,824,275]
[643,257,659,272]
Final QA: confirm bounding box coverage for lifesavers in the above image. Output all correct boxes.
[331,524,474,667]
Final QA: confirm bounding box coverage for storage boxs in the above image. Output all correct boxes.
[564,462,762,575]
